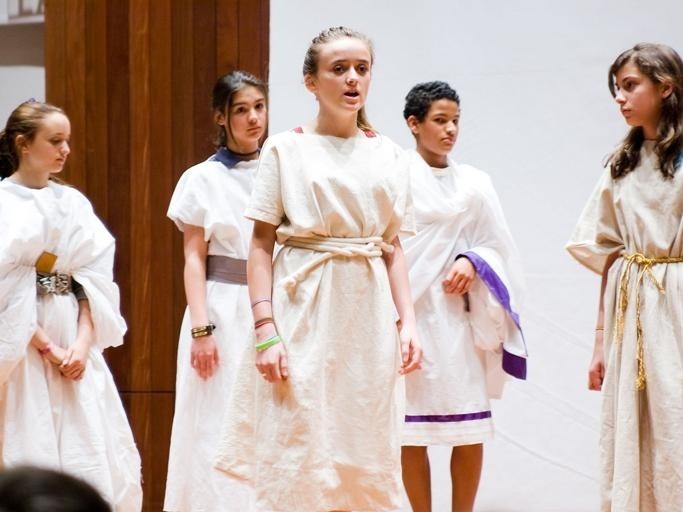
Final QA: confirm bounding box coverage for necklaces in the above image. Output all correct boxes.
[225,146,258,156]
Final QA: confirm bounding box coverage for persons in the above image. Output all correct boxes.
[559,40,682,512]
[398,79,532,511]
[239,24,425,512]
[1,96,146,511]
[159,67,287,511]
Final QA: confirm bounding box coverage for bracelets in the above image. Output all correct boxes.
[37,340,54,355]
[250,295,271,308]
[190,324,216,338]
[254,317,273,329]
[595,325,604,330]
[255,335,281,354]
